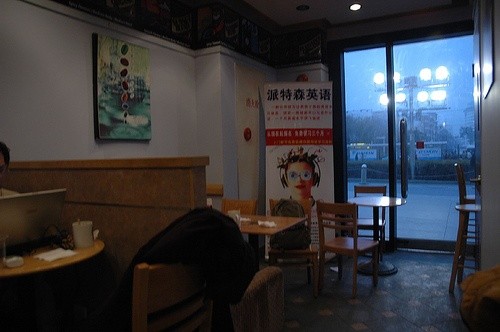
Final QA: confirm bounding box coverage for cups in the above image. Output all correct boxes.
[72,221,94,247]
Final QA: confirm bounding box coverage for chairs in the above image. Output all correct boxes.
[132,263,214,332]
[228,266,284,332]
[222,198,257,215]
[348,185,386,261]
[316,200,380,299]
[269,198,313,285]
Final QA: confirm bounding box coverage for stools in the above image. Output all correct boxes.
[448,161,483,294]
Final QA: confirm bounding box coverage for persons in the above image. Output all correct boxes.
[0,141,23,197]
[283,152,333,247]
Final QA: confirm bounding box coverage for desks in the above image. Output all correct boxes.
[241,214,308,264]
[348,196,408,276]
[0,238,105,332]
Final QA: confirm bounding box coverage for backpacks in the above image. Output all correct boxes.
[270,198,311,250]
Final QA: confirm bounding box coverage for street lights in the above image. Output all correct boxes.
[370,65,449,178]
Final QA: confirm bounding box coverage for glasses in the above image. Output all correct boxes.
[287,169,313,181]
